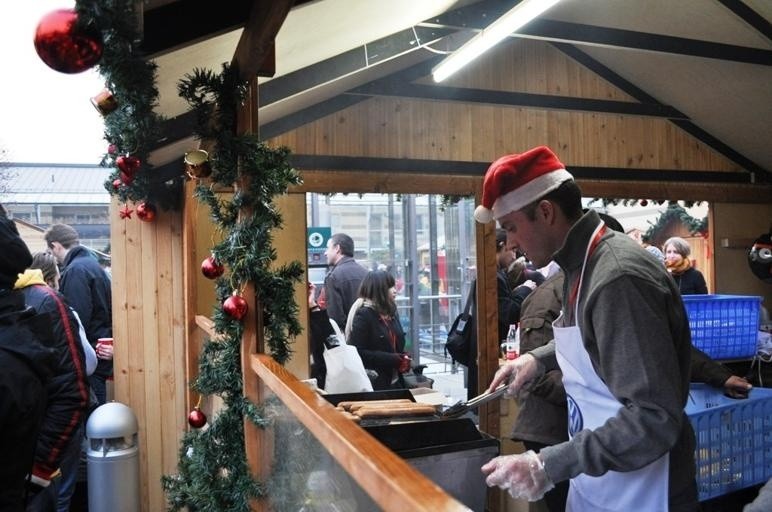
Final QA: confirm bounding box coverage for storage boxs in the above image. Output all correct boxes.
[684,382,772,501]
[680,294,764,361]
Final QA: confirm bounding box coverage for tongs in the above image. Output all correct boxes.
[440,385,511,420]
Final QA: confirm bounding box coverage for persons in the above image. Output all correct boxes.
[31,251,99,511]
[1,204,56,512]
[46,222,114,412]
[464,229,538,398]
[13,235,89,511]
[324,233,370,335]
[345,270,412,390]
[474,145,698,510]
[510,209,751,511]
[663,237,708,295]
[309,283,340,390]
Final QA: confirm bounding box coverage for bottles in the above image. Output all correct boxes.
[516,322,521,358]
[506,324,516,362]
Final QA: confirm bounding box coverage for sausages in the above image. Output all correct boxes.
[338,400,413,408]
[351,402,417,409]
[360,403,434,409]
[360,407,436,418]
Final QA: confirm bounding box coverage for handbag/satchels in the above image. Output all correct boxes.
[443,279,478,371]
[324,318,374,394]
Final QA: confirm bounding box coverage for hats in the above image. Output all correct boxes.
[475,146,574,225]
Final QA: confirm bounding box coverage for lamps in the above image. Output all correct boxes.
[430,0,560,87]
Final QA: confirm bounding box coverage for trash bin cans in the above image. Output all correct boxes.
[85,400,140,511]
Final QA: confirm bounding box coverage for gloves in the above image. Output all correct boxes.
[25,463,55,501]
[482,449,554,509]
[486,352,538,398]
